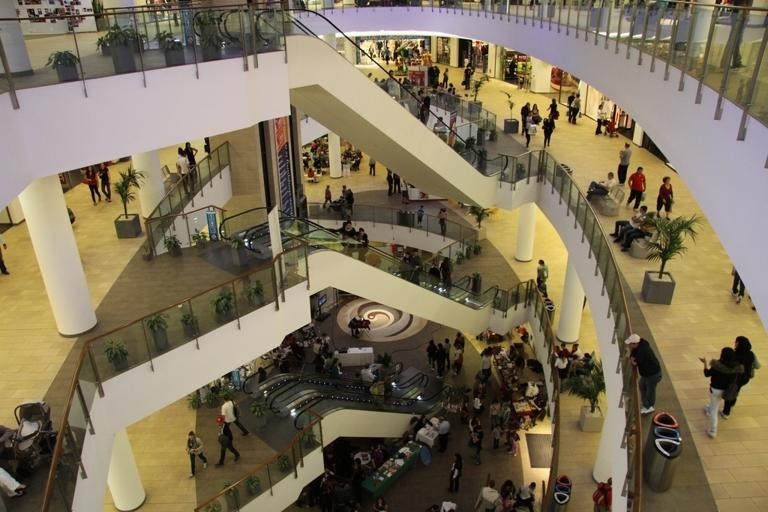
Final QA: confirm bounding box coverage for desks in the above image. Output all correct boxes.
[334,347,374,367]
[362,441,423,499]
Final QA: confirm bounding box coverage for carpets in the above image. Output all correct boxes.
[525,433,552,467]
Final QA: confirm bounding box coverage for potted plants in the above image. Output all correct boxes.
[224,481,241,510]
[94,276,300,374]
[232,234,245,266]
[274,454,295,477]
[641,213,708,306]
[457,252,463,264]
[96,20,146,77]
[193,230,209,252]
[166,235,183,259]
[468,73,487,118]
[470,205,489,242]
[155,27,188,70]
[504,90,519,134]
[186,379,236,410]
[566,353,604,437]
[113,168,146,238]
[475,243,481,254]
[201,20,227,63]
[249,401,268,428]
[247,473,260,496]
[299,425,316,449]
[470,270,482,293]
[45,46,83,84]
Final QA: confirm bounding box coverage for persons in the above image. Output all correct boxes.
[311,441,387,510]
[552,343,594,381]
[624,334,662,414]
[409,326,536,510]
[176,142,199,194]
[186,374,248,477]
[302,142,451,295]
[595,104,603,135]
[0,467,28,511]
[0,235,11,276]
[731,266,745,304]
[85,164,111,207]
[586,142,673,251]
[522,92,583,150]
[537,261,549,294]
[509,57,518,79]
[367,40,482,131]
[592,478,613,510]
[256,328,379,390]
[698,336,761,439]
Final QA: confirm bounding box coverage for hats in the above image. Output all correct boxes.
[624,334,640,345]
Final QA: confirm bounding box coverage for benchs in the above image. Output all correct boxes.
[629,237,653,260]
[160,165,185,194]
[592,186,625,217]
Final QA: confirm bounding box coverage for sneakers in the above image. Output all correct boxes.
[624,203,671,222]
[730,292,756,312]
[187,431,249,480]
[609,231,629,253]
[520,123,555,149]
[640,406,656,415]
[568,118,603,136]
[703,403,730,439]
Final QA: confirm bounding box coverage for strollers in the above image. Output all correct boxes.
[326,195,344,213]
[7,399,57,476]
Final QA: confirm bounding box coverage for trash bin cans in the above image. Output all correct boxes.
[645,411,683,494]
[553,474,572,511]
